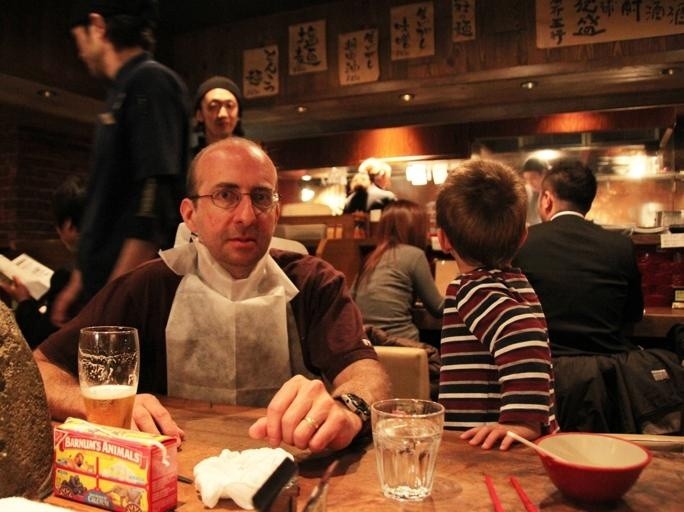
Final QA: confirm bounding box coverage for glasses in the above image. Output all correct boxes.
[189,188,280,209]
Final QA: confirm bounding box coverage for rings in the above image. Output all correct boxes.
[304,416,317,431]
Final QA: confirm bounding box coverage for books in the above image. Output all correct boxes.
[0,253,56,301]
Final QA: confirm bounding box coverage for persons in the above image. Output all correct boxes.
[189,75,244,155]
[350,198,445,341]
[434,156,562,452]
[521,156,550,227]
[45,0,190,329]
[512,157,645,356]
[31,137,396,463]
[342,156,398,214]
[0,168,95,350]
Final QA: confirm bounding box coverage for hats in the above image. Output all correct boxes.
[193,76,245,112]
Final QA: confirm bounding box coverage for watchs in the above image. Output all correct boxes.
[333,392,374,455]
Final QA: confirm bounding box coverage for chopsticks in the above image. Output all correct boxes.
[483,472,539,511]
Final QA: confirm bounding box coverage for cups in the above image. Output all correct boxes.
[77,325,142,431]
[369,397,446,501]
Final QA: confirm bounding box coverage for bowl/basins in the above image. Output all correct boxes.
[535,433,654,502]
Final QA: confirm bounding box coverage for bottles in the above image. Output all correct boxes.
[352,209,367,239]
